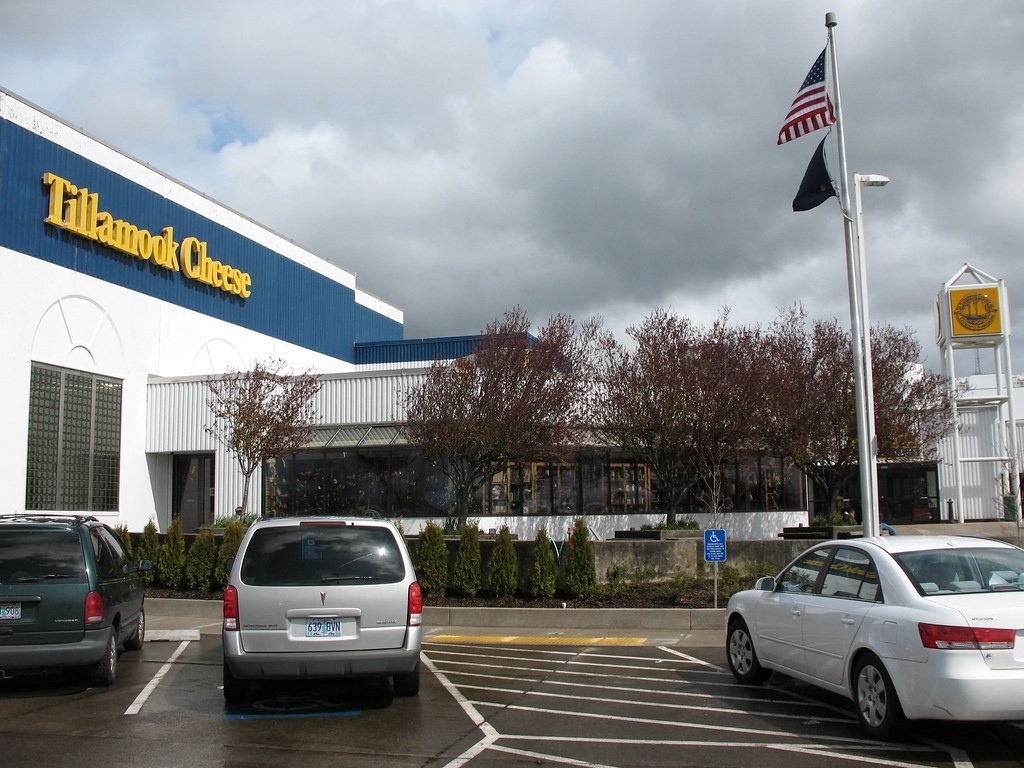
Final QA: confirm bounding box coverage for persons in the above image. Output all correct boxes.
[768,482,779,510]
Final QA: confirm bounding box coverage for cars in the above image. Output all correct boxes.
[723,532,1023,740]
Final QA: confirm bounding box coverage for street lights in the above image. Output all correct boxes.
[852,171,891,533]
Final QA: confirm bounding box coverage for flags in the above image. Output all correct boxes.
[792,130,840,212]
[777,43,837,146]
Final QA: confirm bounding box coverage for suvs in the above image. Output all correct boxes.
[0,512,153,688]
[222,510,425,702]
